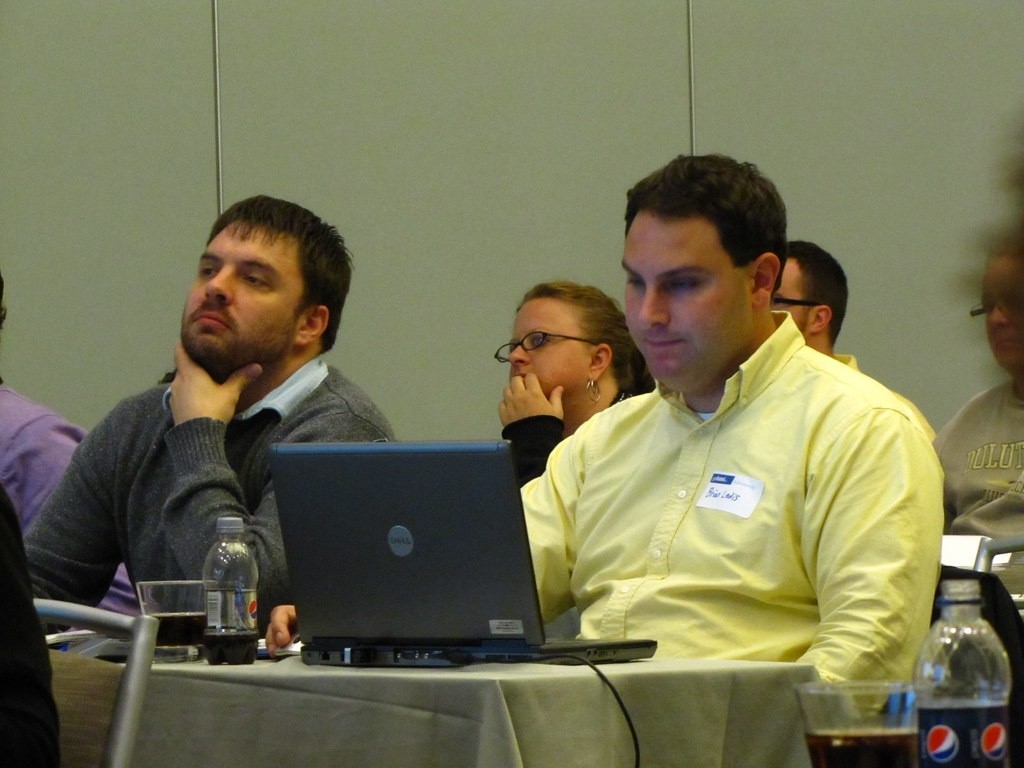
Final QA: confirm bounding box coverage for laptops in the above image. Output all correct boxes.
[269,440,658,665]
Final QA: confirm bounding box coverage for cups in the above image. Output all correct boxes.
[135,579,205,665]
[794,678,918,768]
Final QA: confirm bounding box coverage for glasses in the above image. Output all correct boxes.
[493,331,598,363]
[970,297,1024,320]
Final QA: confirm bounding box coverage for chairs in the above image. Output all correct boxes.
[32,599,160,768]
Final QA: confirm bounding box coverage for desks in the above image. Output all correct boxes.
[93,648,820,768]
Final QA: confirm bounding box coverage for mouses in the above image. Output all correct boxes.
[275,632,307,657]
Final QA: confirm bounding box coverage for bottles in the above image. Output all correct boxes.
[911,578,1014,768]
[201,517,259,665]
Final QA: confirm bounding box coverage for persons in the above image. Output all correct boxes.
[936,221,1024,600]
[771,239,860,375]
[494,282,656,489]
[0,484,60,768]
[263,152,946,715]
[22,194,402,649]
[0,270,141,641]
